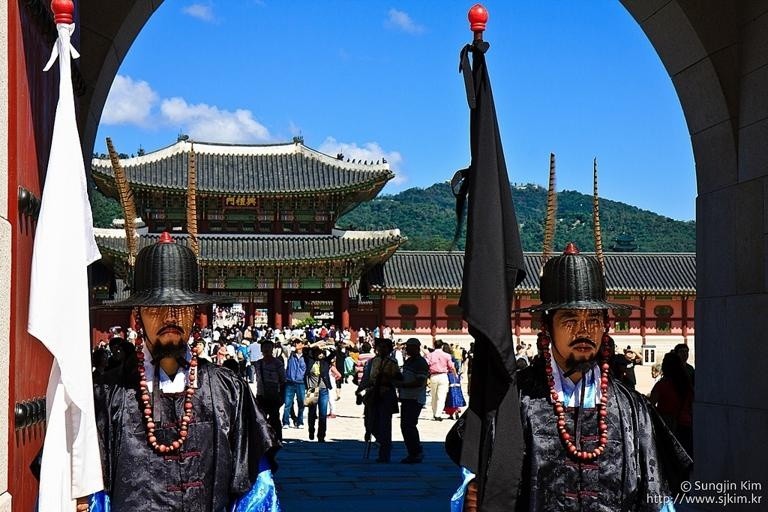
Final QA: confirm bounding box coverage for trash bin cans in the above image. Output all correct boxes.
[641,345,657,366]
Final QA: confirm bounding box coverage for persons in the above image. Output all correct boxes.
[672,344,694,394]
[648,362,663,381]
[619,344,641,391]
[72,232,284,510]
[649,351,694,461]
[445,244,694,512]
[91,310,536,465]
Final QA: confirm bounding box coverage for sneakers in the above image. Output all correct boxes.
[434,414,458,421]
[401,455,422,463]
[310,433,324,442]
[283,424,304,429]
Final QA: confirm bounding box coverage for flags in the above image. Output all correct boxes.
[443,37,527,512]
[25,21,105,512]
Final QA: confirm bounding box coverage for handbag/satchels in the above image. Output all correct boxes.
[303,388,320,407]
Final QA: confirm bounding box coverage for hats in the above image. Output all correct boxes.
[511,244,644,313]
[90,231,235,310]
